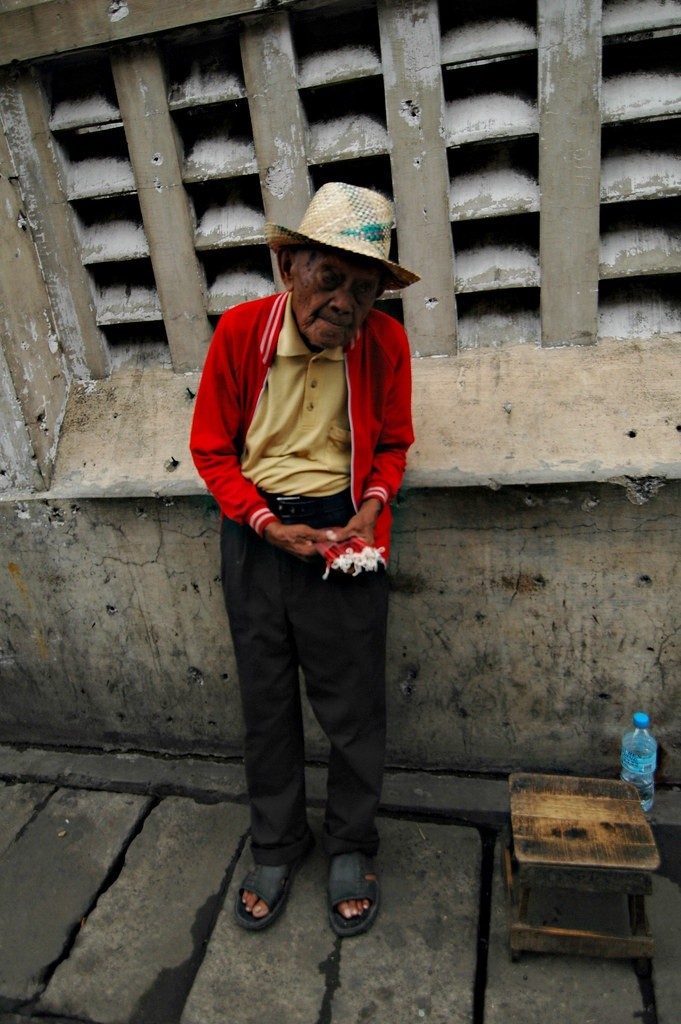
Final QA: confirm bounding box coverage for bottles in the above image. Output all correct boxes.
[619,711,657,811]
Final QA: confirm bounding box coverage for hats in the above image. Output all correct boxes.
[264,182,421,290]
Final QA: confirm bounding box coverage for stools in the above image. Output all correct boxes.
[504,771,658,978]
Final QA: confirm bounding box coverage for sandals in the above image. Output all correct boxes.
[235,855,307,930]
[328,852,380,936]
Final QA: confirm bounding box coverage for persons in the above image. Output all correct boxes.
[191,181,415,941]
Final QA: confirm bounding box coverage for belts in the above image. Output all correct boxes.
[265,491,349,519]
